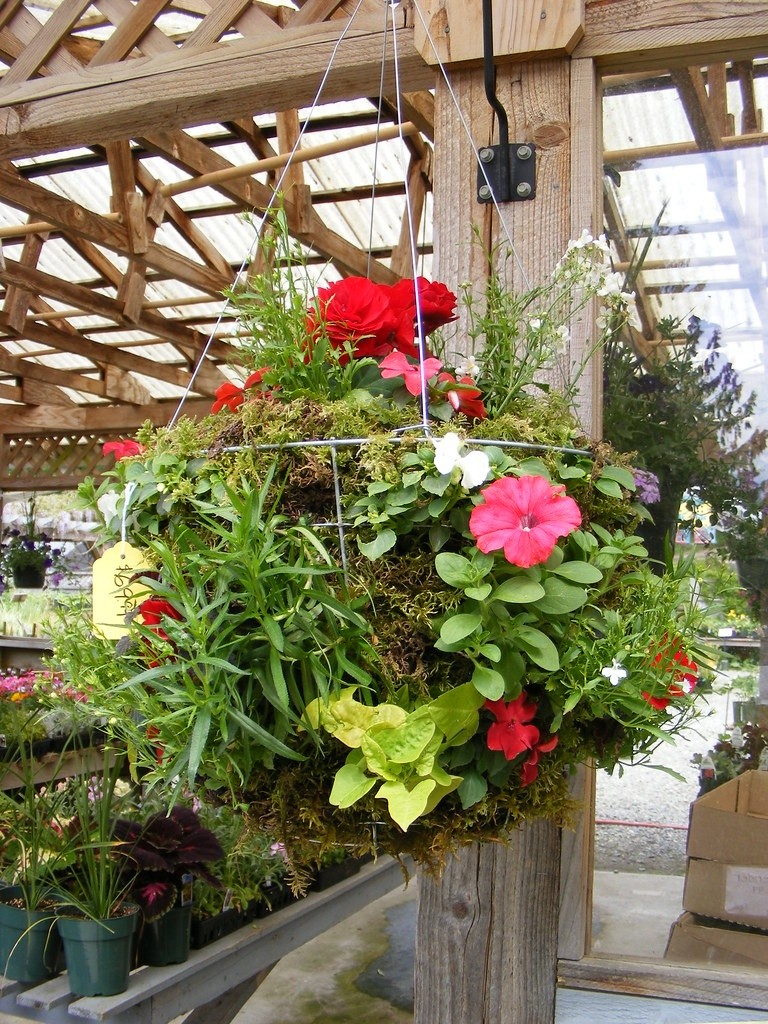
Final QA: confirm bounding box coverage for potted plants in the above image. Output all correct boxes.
[42,723,156,996]
[0,698,72,982]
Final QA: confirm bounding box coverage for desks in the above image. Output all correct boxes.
[0,850,415,1024]
[0,742,121,793]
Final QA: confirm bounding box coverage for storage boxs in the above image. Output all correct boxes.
[683,769,768,931]
[664,912,768,971]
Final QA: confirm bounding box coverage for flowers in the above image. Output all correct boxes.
[48,272,745,831]
[0,667,308,920]
[691,724,768,796]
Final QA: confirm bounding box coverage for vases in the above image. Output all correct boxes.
[133,852,361,967]
